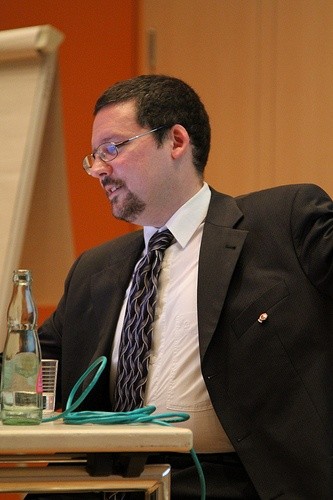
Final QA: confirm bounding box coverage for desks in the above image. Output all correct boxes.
[1,409,194,500]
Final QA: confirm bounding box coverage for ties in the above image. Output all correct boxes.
[109,228,175,413]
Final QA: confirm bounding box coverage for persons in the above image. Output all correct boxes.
[0,75,332,500]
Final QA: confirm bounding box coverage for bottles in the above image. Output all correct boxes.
[0,270,44,425]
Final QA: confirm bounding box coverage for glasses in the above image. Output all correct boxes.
[83,125,166,176]
